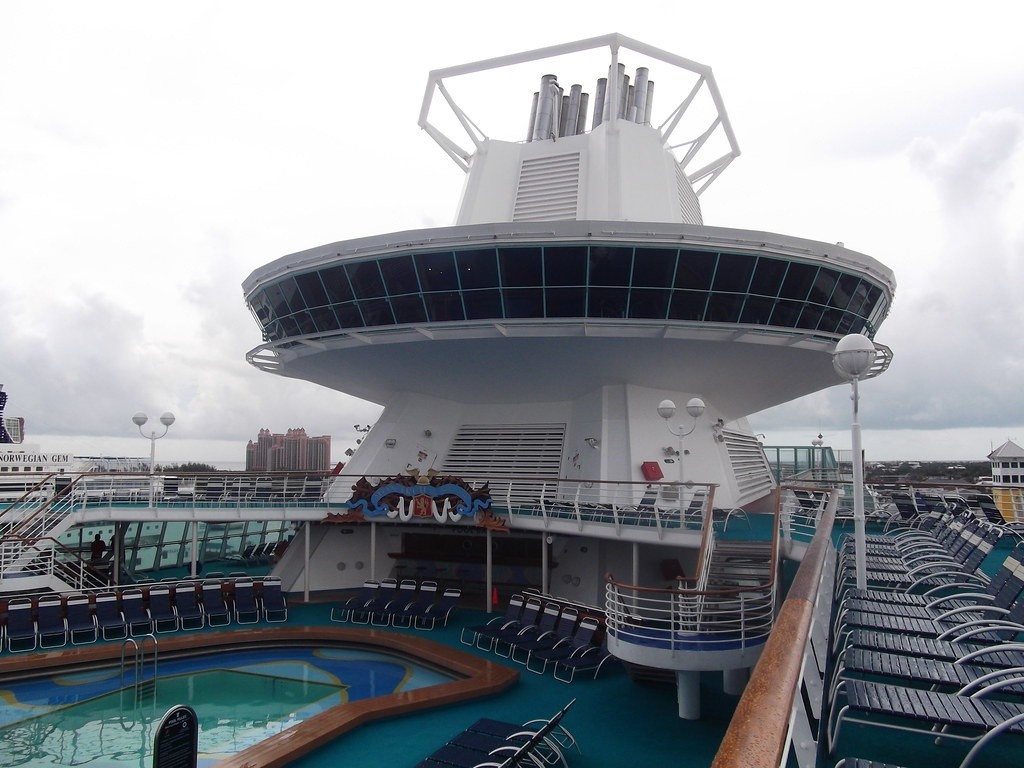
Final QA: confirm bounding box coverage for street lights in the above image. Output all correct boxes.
[832,333,877,591]
[132,410,176,508]
[657,396,708,530]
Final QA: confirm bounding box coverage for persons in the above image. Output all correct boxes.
[91,534,107,561]
[111,535,115,554]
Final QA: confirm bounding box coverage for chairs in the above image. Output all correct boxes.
[528,484,751,533]
[158,476,330,506]
[793,484,1024,768]
[371,580,417,626]
[476,597,542,652]
[460,593,525,646]
[552,633,612,684]
[391,582,438,628]
[0,540,292,654]
[527,618,600,675]
[511,607,579,665]
[414,588,462,631]
[493,602,561,658]
[411,699,582,768]
[352,577,397,624]
[330,579,379,622]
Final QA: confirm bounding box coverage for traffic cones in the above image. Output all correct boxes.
[492,586,499,606]
